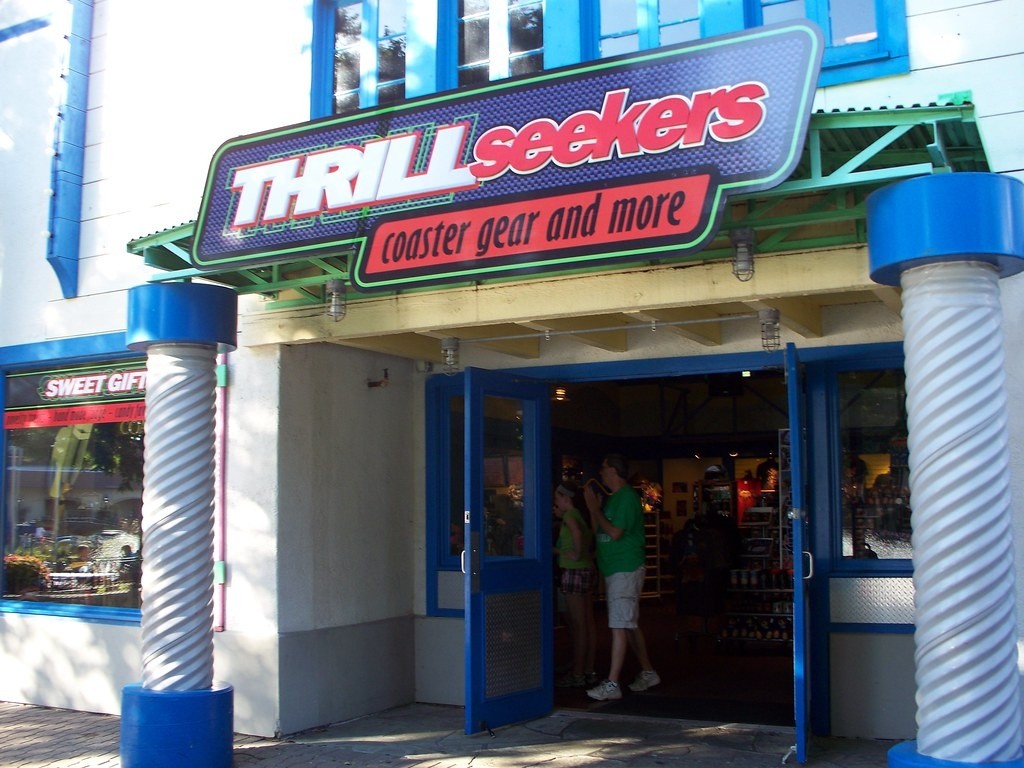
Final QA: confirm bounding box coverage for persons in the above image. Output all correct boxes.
[549,482,597,686]
[584,453,661,701]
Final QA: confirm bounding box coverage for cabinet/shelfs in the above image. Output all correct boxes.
[590,427,913,653]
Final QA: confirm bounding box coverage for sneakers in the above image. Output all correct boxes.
[628,668,660,692]
[587,680,623,700]
[554,669,586,688]
[585,668,601,683]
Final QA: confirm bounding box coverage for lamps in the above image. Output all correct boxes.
[728,226,757,282]
[440,338,460,377]
[758,310,781,355]
[324,279,348,323]
